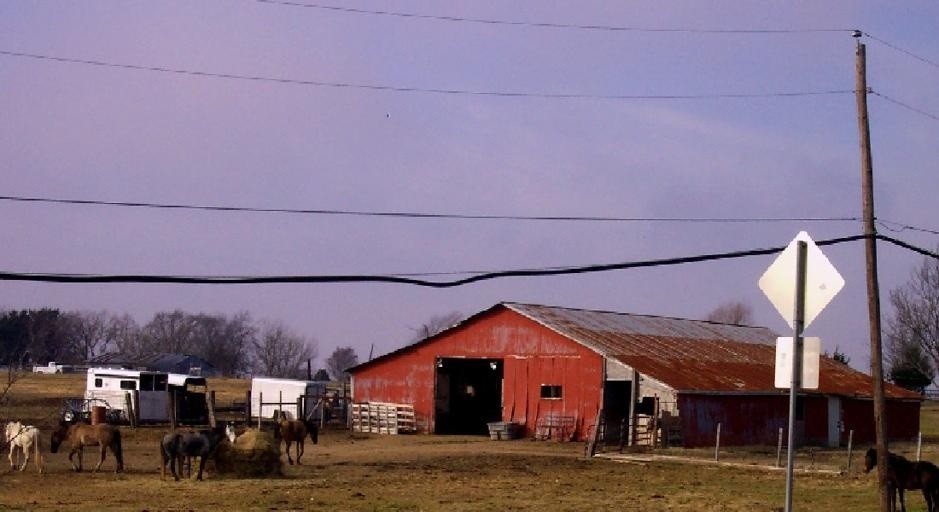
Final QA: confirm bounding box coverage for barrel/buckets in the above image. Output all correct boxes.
[92,406,105,425]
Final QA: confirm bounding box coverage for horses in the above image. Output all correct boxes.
[5,421,44,474]
[160,420,237,481]
[862,445,939,512]
[50,421,124,478]
[274,418,319,466]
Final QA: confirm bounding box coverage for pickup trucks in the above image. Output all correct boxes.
[32,361,73,375]
